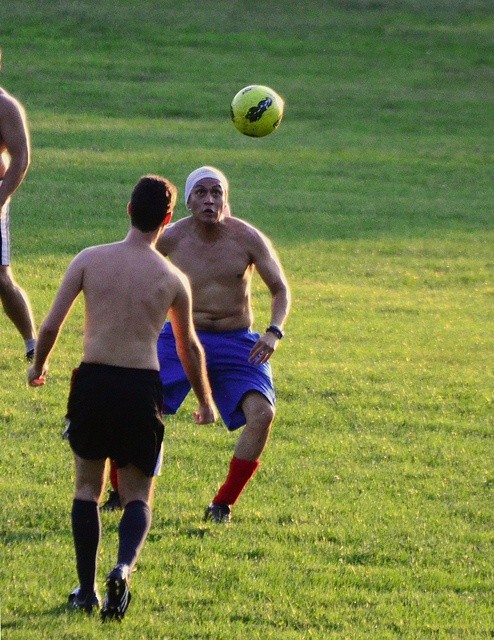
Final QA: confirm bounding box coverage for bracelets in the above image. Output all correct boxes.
[266,325,283,339]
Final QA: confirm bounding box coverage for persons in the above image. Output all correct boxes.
[27,173,219,620]
[0,91,39,362]
[156,166,290,524]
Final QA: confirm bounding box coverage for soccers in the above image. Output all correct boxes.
[230,84,283,138]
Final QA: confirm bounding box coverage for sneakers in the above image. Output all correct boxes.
[206,502,231,524]
[100,578,132,622]
[68,587,100,613]
